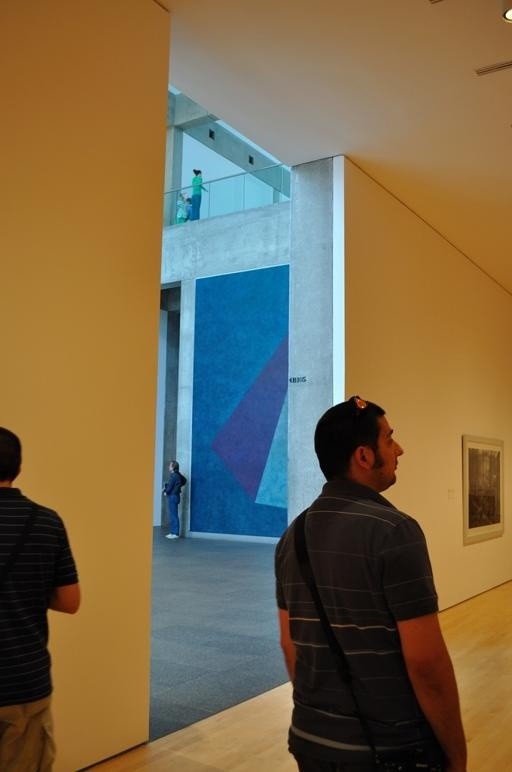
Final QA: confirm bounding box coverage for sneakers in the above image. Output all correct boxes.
[164,532,180,539]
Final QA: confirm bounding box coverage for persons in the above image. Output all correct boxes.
[0,428,80,772]
[273,395,467,772]
[163,461,187,540]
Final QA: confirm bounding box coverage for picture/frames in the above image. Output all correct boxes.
[460,434,507,545]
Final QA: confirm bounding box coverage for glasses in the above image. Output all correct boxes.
[347,392,368,445]
